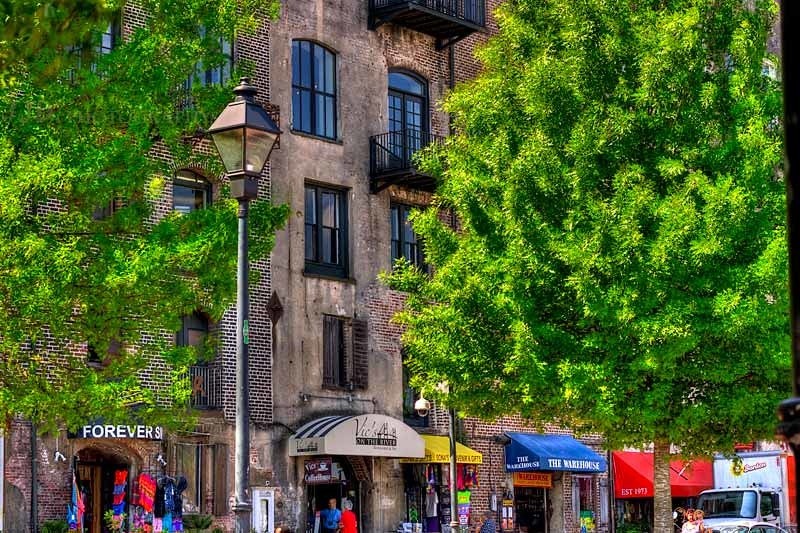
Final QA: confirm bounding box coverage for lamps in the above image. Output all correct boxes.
[572,476,581,526]
[598,476,610,532]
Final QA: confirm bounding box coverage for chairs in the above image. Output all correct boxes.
[717,499,769,515]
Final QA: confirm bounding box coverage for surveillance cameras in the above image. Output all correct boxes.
[415,398,430,417]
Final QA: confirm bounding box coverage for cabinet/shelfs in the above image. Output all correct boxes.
[438,484,452,525]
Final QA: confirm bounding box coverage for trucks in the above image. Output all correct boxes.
[695,450,796,532]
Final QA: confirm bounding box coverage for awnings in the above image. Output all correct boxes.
[400,435,482,465]
[503,430,606,472]
[289,412,426,459]
[612,450,714,499]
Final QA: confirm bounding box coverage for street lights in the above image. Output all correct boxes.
[204,77,284,533]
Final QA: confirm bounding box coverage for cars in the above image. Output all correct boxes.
[704,522,789,533]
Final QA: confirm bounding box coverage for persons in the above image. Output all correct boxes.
[320,498,341,533]
[690,509,705,533]
[682,508,712,533]
[480,511,496,533]
[338,500,358,533]
[476,515,486,533]
